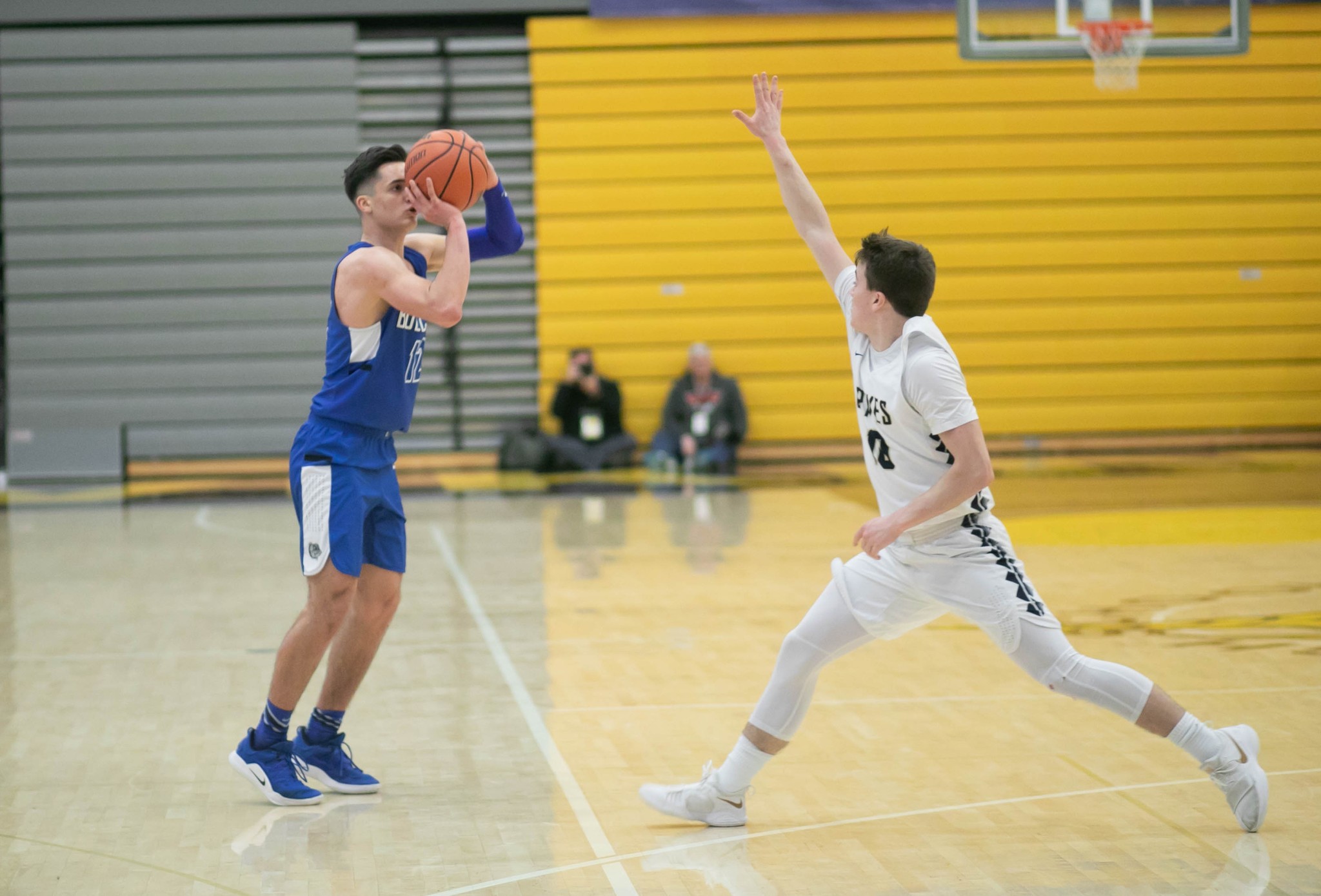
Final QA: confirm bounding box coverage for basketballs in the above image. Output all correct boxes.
[404,127,490,213]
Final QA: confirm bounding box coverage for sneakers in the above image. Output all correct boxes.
[1200,725,1267,833]
[229,729,323,806]
[292,726,381,794]
[641,761,745,827]
[1204,834,1270,895]
[641,826,747,874]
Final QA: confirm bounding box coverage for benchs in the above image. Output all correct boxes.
[0,0,1321,500]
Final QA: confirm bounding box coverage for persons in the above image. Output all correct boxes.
[643,825,1272,896]
[229,145,527,806]
[651,482,750,573]
[548,349,635,475]
[230,790,377,896]
[636,72,1266,834]
[648,344,750,477]
[554,488,642,582]
